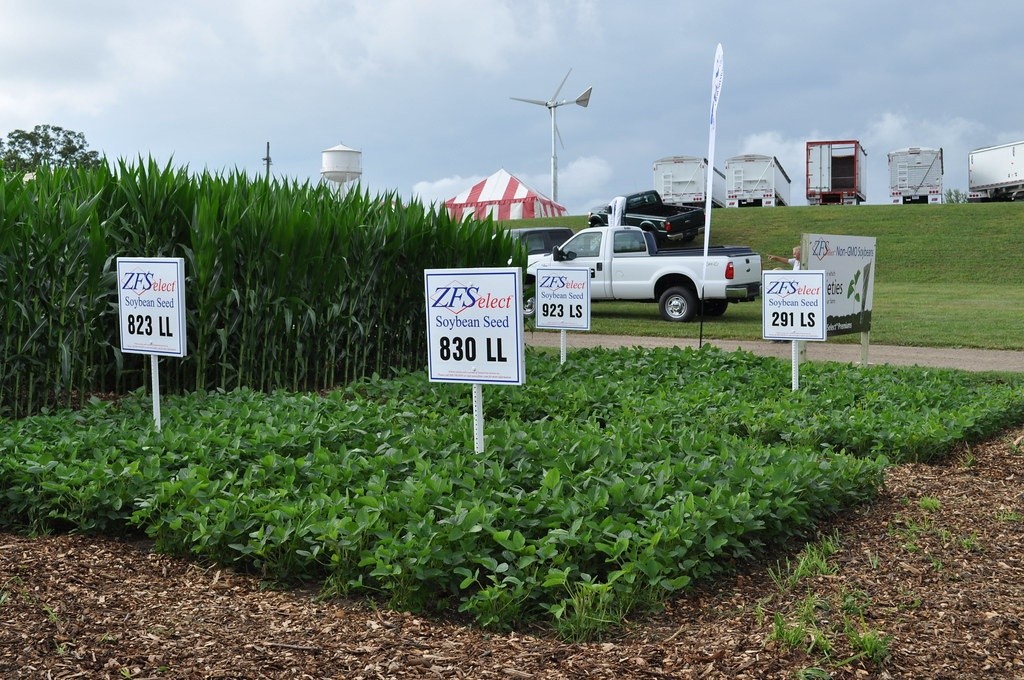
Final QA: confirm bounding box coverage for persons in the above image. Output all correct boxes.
[766,247,802,271]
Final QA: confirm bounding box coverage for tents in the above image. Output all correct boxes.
[443,168,567,223]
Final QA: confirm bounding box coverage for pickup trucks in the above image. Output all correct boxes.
[588,190,705,248]
[523,226,762,323]
[491,228,575,267]
